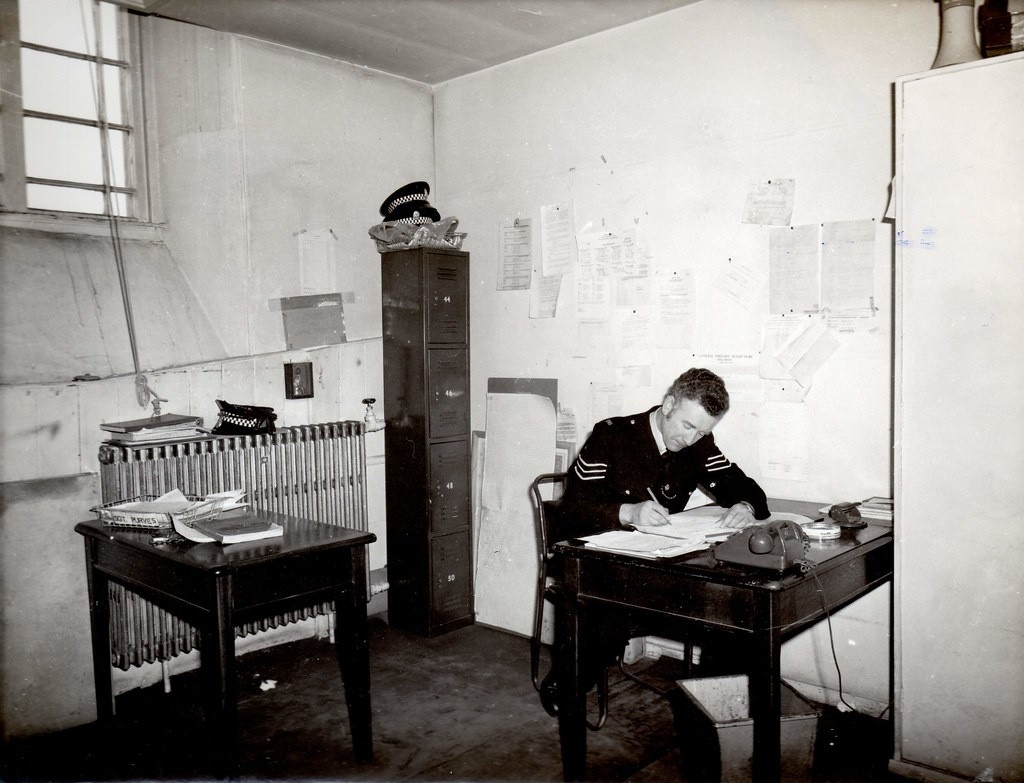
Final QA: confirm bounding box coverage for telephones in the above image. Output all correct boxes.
[712,518,811,571]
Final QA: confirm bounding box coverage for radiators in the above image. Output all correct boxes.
[98,420,372,671]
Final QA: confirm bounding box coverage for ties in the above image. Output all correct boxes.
[662,452,675,473]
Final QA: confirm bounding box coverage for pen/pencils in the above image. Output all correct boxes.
[705,531,734,538]
[647,487,673,525]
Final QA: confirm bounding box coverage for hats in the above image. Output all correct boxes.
[211,399,278,436]
[380,181,441,227]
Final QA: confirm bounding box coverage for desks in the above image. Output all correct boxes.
[74,506,377,783]
[552,498,893,783]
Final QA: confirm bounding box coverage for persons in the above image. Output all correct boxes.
[539,367,770,717]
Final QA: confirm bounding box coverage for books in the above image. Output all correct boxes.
[99,412,217,449]
[194,506,285,545]
[819,495,893,519]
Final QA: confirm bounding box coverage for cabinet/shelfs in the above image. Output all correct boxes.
[381,247,475,639]
[889,51,1024,783]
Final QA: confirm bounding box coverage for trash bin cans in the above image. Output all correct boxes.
[677,671,824,783]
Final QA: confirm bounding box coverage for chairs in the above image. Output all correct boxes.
[531,472,693,732]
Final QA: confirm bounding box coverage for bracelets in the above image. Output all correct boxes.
[742,501,755,514]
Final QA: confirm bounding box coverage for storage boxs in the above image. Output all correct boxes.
[674,675,822,783]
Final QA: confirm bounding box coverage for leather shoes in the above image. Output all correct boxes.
[540,663,567,718]
